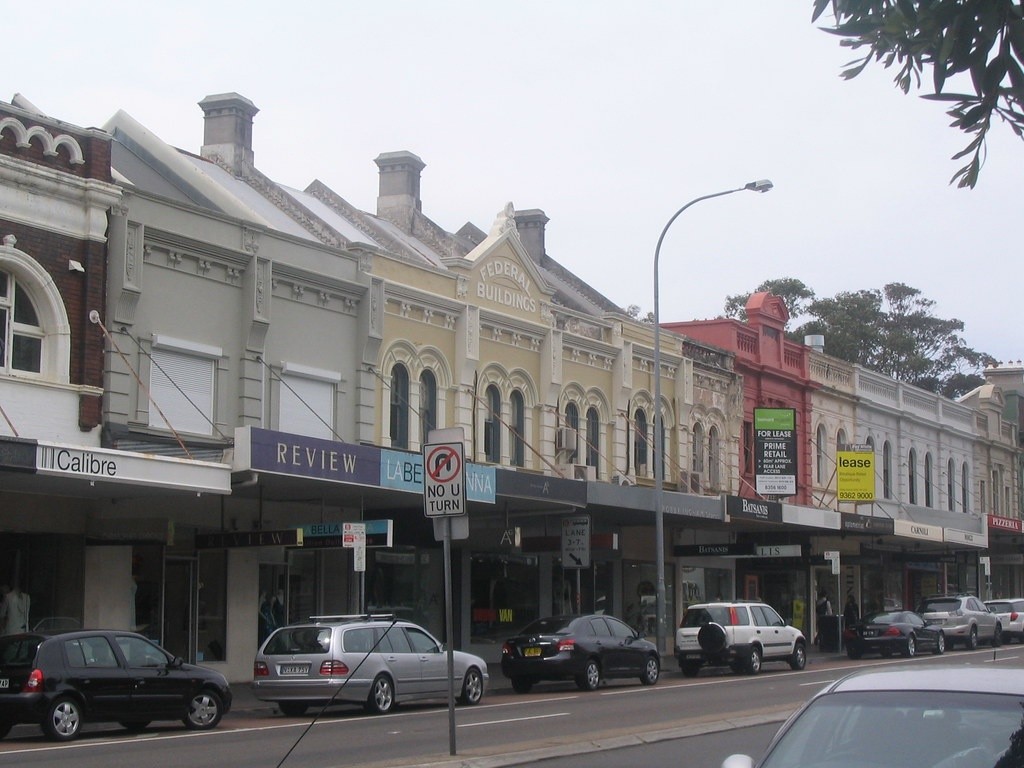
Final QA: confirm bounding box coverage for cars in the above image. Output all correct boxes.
[845,610,946,659]
[501,614,660,694]
[721,662,1024,768]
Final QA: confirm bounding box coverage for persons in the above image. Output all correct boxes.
[844,596,859,626]
[818,591,831,618]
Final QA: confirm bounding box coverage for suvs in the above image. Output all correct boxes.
[915,593,1002,650]
[675,600,807,677]
[983,598,1024,644]
[252,614,489,715]
[0,628,233,741]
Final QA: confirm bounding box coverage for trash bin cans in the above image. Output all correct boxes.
[817,614,844,653]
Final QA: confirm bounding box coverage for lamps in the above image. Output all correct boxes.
[68,260,85,273]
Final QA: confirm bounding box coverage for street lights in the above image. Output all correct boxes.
[653,179,774,664]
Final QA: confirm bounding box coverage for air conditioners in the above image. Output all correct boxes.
[556,426,576,451]
